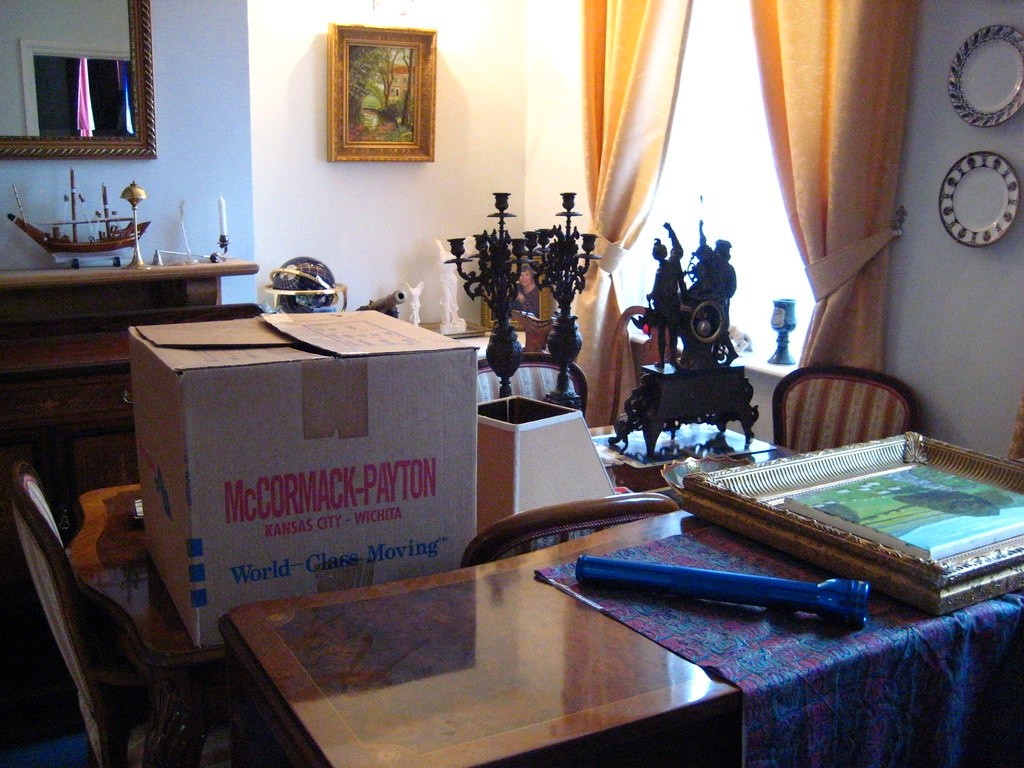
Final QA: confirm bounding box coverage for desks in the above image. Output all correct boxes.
[216,509,1024,768]
[66,422,799,768]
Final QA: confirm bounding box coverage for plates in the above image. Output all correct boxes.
[660,455,755,496]
[939,152,1020,248]
[948,25,1024,127]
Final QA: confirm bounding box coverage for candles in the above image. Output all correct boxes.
[217,194,228,235]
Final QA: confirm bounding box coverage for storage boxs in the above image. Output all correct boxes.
[126,308,481,650]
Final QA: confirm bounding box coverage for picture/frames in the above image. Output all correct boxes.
[684,431,1024,590]
[327,21,438,164]
[675,487,1024,617]
[480,254,549,331]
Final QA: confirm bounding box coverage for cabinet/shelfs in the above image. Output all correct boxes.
[0,263,261,753]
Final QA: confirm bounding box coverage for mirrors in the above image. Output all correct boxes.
[0,0,158,160]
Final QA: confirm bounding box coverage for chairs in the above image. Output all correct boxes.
[604,304,671,426]
[7,459,233,768]
[477,351,589,419]
[772,364,923,454]
[460,492,679,570]
